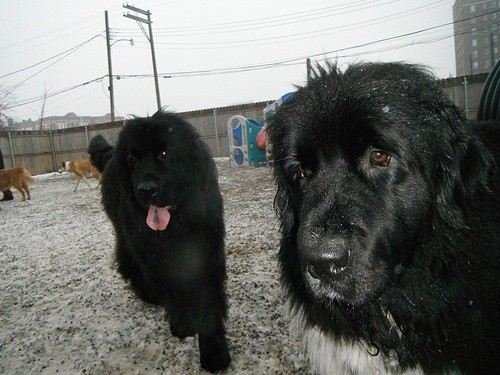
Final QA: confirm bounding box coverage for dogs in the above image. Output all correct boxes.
[58,160,102,192]
[0,167,35,202]
[261,58,500,375]
[88,105,231,373]
